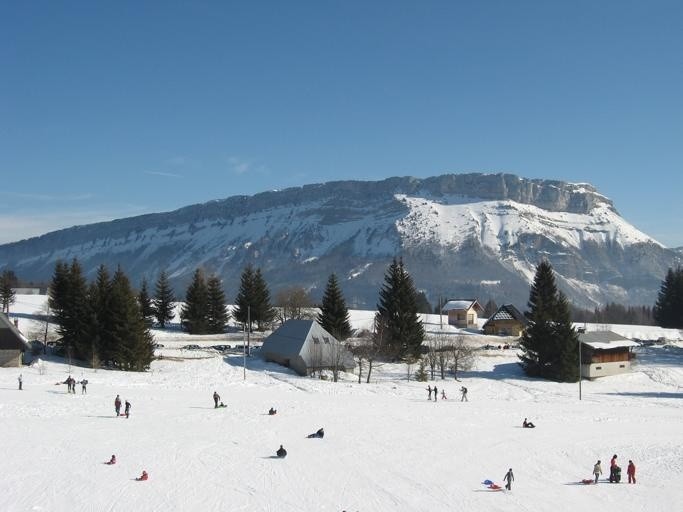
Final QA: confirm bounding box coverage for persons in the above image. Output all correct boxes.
[591,460,603,484]
[17,373,24,391]
[80,376,88,394]
[115,396,123,416]
[609,454,620,484]
[523,417,535,428]
[70,378,76,395]
[268,407,277,415]
[124,399,132,418]
[425,384,433,400]
[440,389,447,399]
[116,394,121,402]
[212,391,221,408]
[307,426,324,438]
[627,459,637,484]
[276,445,287,458]
[503,468,516,491]
[62,373,73,393]
[105,454,116,465]
[432,386,438,402]
[135,470,149,481]
[458,385,469,402]
[219,402,228,408]
